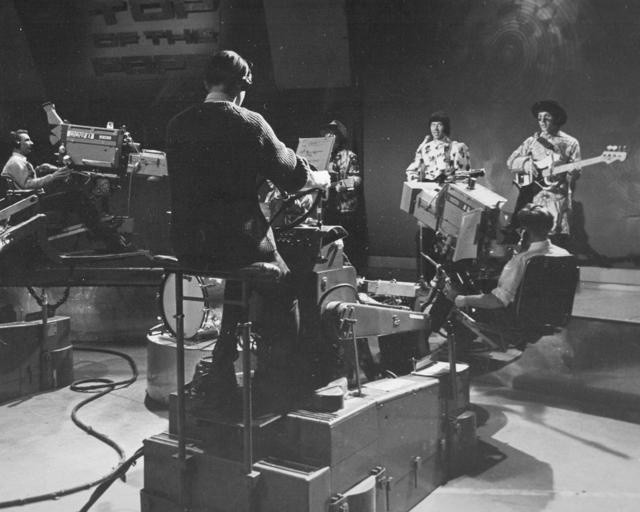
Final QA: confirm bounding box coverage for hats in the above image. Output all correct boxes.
[532,100,567,125]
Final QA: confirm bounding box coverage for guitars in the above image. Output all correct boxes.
[512,146,627,190]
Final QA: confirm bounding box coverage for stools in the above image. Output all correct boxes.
[151,254,283,475]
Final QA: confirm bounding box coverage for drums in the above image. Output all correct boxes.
[157,272,224,342]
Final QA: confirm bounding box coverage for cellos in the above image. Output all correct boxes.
[407,169,484,183]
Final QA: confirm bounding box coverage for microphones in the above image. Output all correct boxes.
[454,169,485,178]
[535,137,560,153]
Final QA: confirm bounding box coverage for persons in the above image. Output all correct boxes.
[405,110,472,282]
[506,100,582,230]
[420,205,573,332]
[322,120,362,225]
[1,129,124,251]
[164,50,330,362]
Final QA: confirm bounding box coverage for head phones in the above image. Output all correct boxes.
[12,139,20,148]
[240,62,254,89]
[519,205,543,237]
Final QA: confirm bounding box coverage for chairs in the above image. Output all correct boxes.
[0,176,91,249]
[452,255,582,353]
